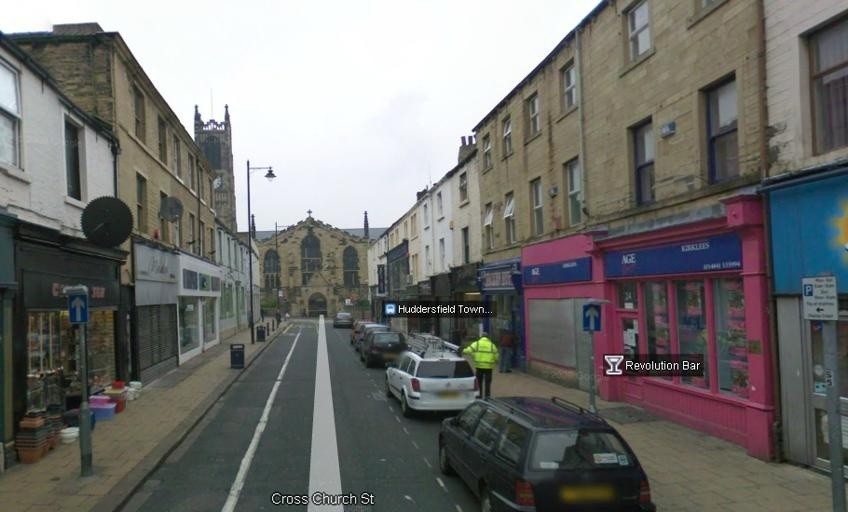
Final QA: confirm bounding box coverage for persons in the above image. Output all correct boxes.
[463,331,499,398]
[261,305,265,321]
[276,308,281,327]
[285,309,289,320]
[302,307,308,318]
[500,330,514,373]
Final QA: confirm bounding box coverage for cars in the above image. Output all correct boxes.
[350,319,409,369]
[333,312,354,329]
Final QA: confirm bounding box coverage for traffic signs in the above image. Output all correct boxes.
[801,273,839,322]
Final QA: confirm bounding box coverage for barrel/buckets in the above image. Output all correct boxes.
[129,381,142,399]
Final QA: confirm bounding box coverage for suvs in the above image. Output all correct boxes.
[437,394,658,511]
[385,345,482,418]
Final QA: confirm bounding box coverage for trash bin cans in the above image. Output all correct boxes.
[256,325,265,342]
[231,344,244,369]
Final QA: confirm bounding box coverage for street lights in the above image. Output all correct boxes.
[246,160,277,346]
